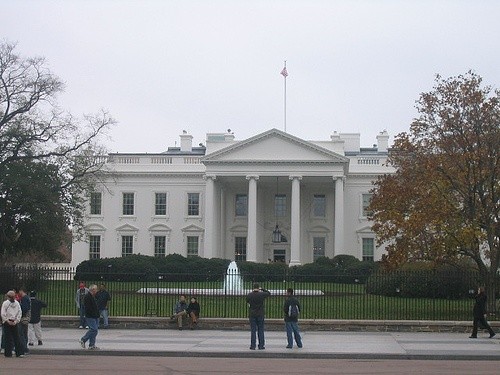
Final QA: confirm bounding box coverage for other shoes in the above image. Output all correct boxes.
[88,346,100,350]
[28,343,33,345]
[38,340,42,345]
[81,340,85,348]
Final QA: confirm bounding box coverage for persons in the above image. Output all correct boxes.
[186,297,200,330]
[1,291,26,358]
[0,289,21,354]
[28,289,47,346]
[81,284,101,351]
[283,288,303,348]
[170,295,188,330]
[75,284,91,329]
[96,284,111,329]
[15,288,31,353]
[469,287,496,339]
[245,284,270,350]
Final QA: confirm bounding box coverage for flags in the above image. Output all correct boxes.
[280,60,289,77]
